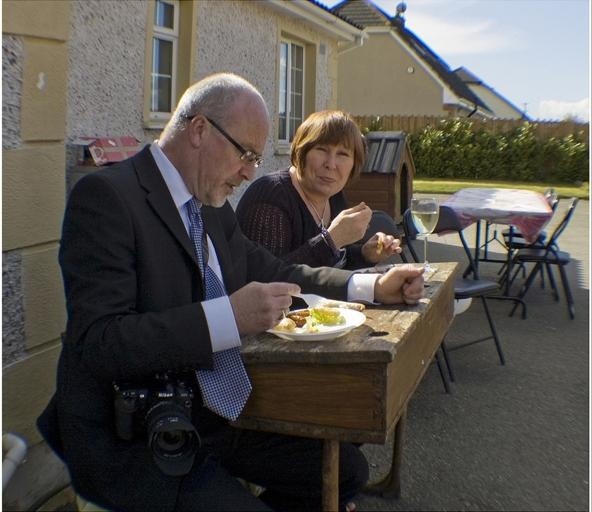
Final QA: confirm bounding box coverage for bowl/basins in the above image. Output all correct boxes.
[294,162,328,228]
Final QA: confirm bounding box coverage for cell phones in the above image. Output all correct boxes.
[110,370,202,478]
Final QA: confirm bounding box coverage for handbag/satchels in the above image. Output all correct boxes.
[321,229,340,257]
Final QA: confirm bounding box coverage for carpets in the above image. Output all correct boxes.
[186,195,252,421]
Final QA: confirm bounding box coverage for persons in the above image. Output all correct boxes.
[235,109,405,512]
[36,72,370,511]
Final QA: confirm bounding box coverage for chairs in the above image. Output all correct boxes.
[346,210,453,394]
[498,189,580,321]
[403,203,507,384]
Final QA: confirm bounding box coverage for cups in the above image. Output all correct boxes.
[185,114,264,168]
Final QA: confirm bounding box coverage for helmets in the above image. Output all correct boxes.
[266,306,366,341]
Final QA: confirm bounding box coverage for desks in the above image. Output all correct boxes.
[437,189,553,321]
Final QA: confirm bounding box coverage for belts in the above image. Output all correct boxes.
[411,197,440,275]
